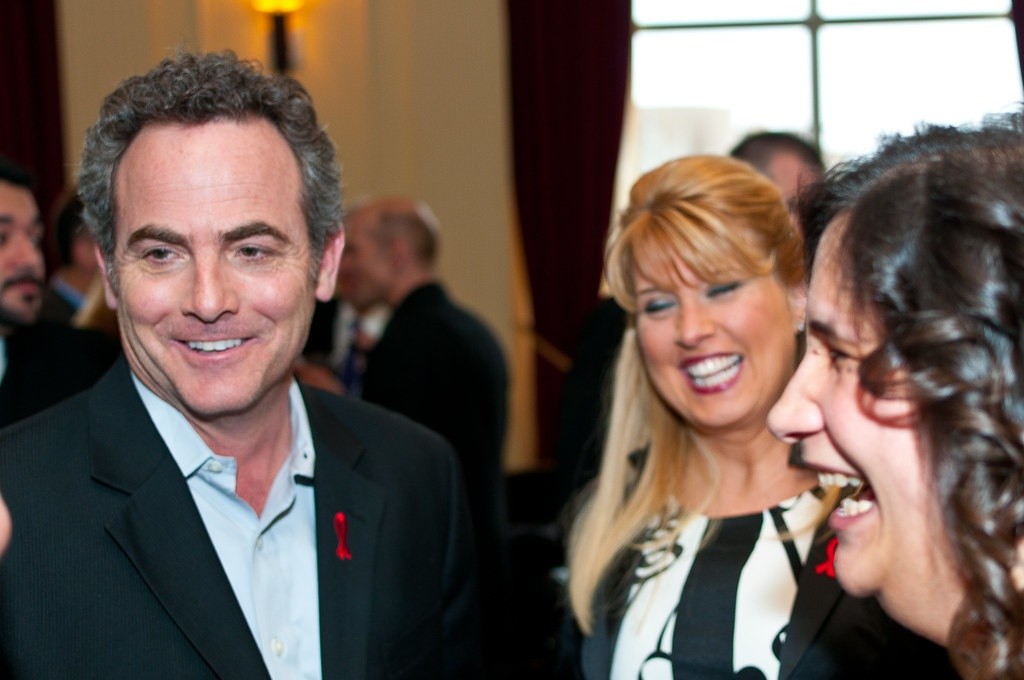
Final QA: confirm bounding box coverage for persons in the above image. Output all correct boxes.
[295,201,507,680]
[732,131,822,228]
[1,180,121,426]
[0,49,503,680]
[766,114,1023,680]
[561,157,962,680]
